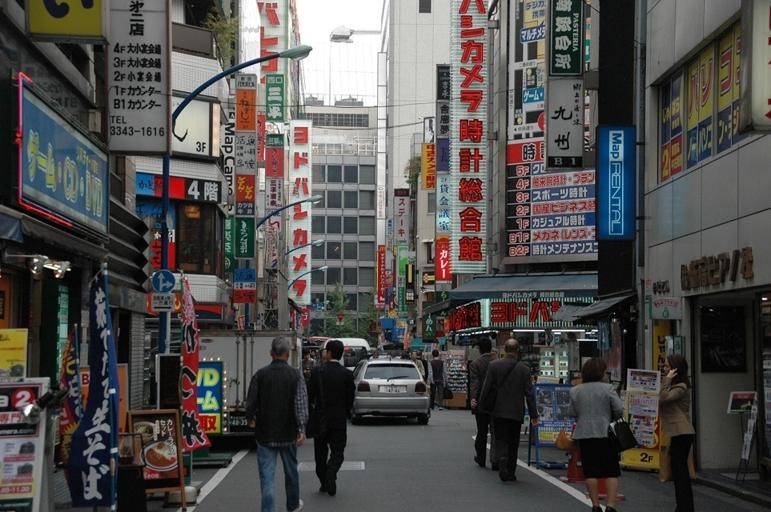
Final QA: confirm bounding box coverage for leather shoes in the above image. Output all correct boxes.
[492,463,517,482]
[474,456,486,468]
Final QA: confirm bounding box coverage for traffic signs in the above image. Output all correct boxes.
[151,292,175,312]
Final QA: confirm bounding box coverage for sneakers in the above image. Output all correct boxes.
[289,499,304,512]
[318,469,337,496]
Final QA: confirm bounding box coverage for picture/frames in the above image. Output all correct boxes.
[127,409,184,494]
[727,391,756,414]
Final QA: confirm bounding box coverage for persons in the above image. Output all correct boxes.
[402,349,429,383]
[658,351,700,512]
[476,338,542,483]
[301,347,328,384]
[467,335,504,472]
[244,339,310,511]
[304,338,357,495]
[565,356,621,511]
[429,349,446,411]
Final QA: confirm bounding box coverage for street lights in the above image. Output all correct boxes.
[147,41,316,395]
[267,239,327,299]
[243,195,327,327]
[287,265,333,290]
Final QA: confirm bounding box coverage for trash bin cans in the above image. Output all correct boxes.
[117,433,147,512]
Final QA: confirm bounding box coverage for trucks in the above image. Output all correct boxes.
[189,330,306,448]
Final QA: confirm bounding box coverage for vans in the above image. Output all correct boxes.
[308,336,433,426]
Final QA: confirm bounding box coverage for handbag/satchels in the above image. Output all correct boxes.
[482,361,519,412]
[305,369,318,440]
[556,412,576,451]
[607,413,639,454]
[659,441,672,483]
[443,382,453,401]
